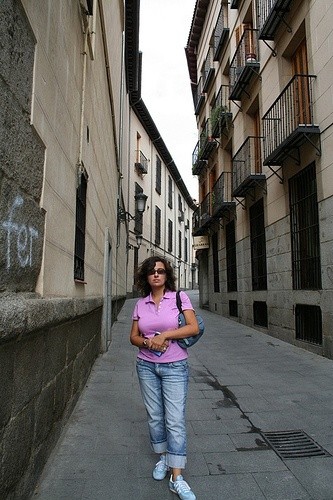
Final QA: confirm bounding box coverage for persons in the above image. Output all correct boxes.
[129,256,199,499]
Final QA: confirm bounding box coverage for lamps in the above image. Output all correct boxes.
[118,192,148,224]
[126,234,143,249]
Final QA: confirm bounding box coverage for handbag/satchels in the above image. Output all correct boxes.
[175,291,205,349]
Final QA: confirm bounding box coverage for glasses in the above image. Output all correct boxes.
[147,268,167,274]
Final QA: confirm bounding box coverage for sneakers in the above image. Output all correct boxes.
[152,453,169,480]
[168,473,197,500]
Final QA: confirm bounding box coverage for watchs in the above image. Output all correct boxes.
[143,339,148,347]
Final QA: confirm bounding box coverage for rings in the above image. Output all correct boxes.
[163,347,167,351]
